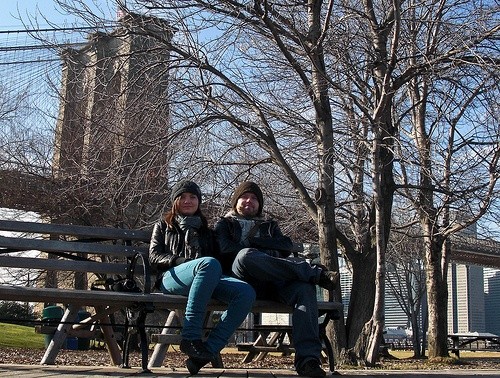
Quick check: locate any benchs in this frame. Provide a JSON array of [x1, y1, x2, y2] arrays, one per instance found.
[[0, 217, 342, 378], [445, 329, 497, 355], [237, 316, 309, 365]]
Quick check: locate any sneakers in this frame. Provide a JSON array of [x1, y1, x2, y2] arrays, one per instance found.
[[320, 268, 340, 290], [298, 360, 326, 378], [180, 339, 215, 360], [185, 357, 210, 376]]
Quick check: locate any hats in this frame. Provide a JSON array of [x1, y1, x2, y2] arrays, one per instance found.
[[232, 181, 263, 212], [172, 181, 201, 202]]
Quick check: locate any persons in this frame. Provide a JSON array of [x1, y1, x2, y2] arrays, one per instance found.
[[214, 181, 341, 378], [148, 179, 257, 377]]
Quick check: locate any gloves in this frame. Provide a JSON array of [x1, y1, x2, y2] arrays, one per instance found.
[[174, 257, 190, 264]]
